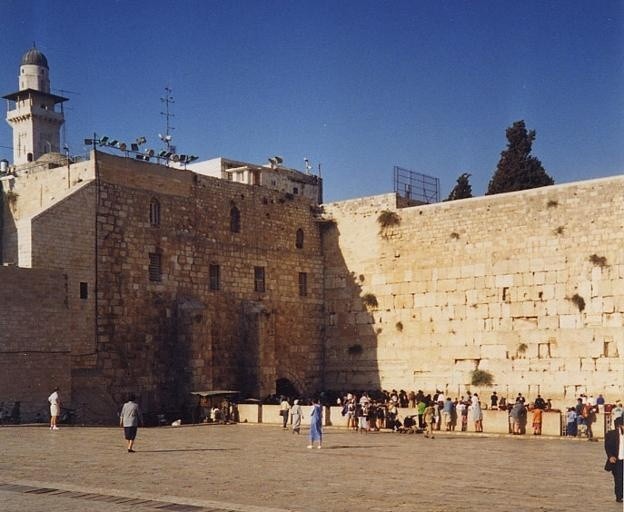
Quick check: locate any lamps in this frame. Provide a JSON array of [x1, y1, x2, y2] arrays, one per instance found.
[[84, 136, 190, 164]]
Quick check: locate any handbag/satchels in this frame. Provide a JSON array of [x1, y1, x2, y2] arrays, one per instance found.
[[280, 410, 283, 416]]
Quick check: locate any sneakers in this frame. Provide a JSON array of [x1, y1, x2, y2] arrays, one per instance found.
[[50, 427, 60, 430], [306, 446, 322, 449], [128, 449, 135, 452]]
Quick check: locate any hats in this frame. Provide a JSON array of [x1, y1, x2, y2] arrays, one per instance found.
[[294, 400, 299, 405]]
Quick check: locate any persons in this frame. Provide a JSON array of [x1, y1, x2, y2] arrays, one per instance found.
[[264, 393, 304, 434], [490, 391, 546, 435], [200, 395, 240, 425], [603, 416, 623, 503], [47, 386, 61, 430], [567, 397, 596, 441], [580, 393, 604, 409], [606, 402, 623, 429], [0, 401, 72, 424], [306, 398, 322, 449], [118, 394, 143, 453], [313, 390, 483, 439]]
